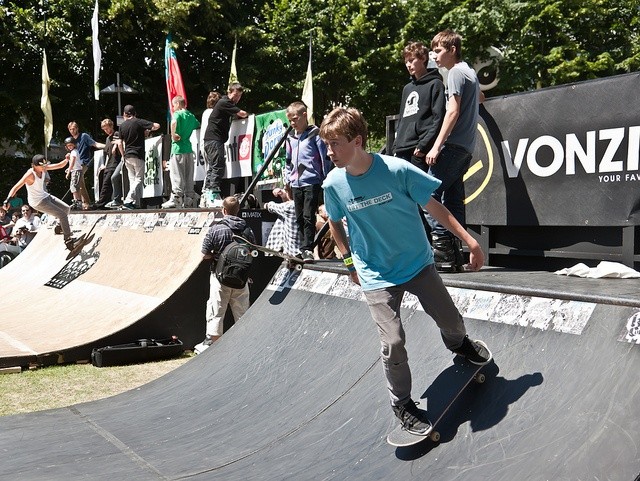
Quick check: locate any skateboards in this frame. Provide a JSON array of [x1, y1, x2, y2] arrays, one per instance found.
[[386, 340, 489, 447], [231, 235, 305, 271], [65, 239, 86, 260], [99, 168, 105, 198], [119, 162, 130, 203], [434, 260, 465, 273]]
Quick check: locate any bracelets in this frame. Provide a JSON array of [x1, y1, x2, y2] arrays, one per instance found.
[[346, 267, 357, 274], [339, 251, 353, 265]]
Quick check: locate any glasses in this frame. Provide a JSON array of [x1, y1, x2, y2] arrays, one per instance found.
[[22, 210, 29, 212]]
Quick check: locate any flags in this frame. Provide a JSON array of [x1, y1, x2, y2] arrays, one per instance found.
[[89, 0, 102, 101], [225, 40, 240, 95], [163, 31, 189, 122], [297, 42, 315, 126], [38, 47, 55, 147]]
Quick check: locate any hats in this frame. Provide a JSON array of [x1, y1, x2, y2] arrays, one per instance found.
[[32, 154, 51, 166], [124, 105, 136, 116]]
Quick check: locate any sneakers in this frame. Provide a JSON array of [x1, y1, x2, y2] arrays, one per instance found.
[[209, 193, 223, 208], [194, 340, 209, 353], [198, 193, 205, 208], [301, 249, 314, 262], [160, 199, 182, 209], [54, 225, 62, 234], [390, 400, 432, 435], [184, 195, 198, 208], [65, 233, 86, 252], [454, 337, 493, 366], [122, 203, 135, 211], [104, 200, 120, 208]]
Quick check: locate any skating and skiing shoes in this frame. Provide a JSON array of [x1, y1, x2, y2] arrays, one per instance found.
[[430, 228, 456, 272], [452, 236, 465, 272], [69, 199, 81, 211]]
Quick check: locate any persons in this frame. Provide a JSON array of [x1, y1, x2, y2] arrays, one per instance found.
[[282, 100, 332, 263], [201, 195, 258, 346], [0, 225, 9, 242], [117, 105, 160, 211], [40, 212, 48, 225], [319, 104, 485, 435], [160, 94, 201, 209], [89, 118, 119, 209], [419, 28, 485, 264], [9, 204, 40, 252], [11, 211, 21, 223], [0, 206, 15, 236], [104, 136, 130, 210], [65, 121, 106, 211], [198, 91, 222, 208], [6, 192, 23, 217], [342, 216, 350, 246], [203, 81, 249, 207], [0, 238, 22, 268], [312, 204, 328, 260], [390, 38, 446, 245], [261, 187, 302, 260], [2, 152, 86, 252], [66, 136, 84, 211]]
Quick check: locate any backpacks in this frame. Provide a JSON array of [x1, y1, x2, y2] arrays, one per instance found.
[[216, 241, 253, 289]]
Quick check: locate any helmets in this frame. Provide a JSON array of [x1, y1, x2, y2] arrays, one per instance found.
[[64, 137, 77, 146]]
